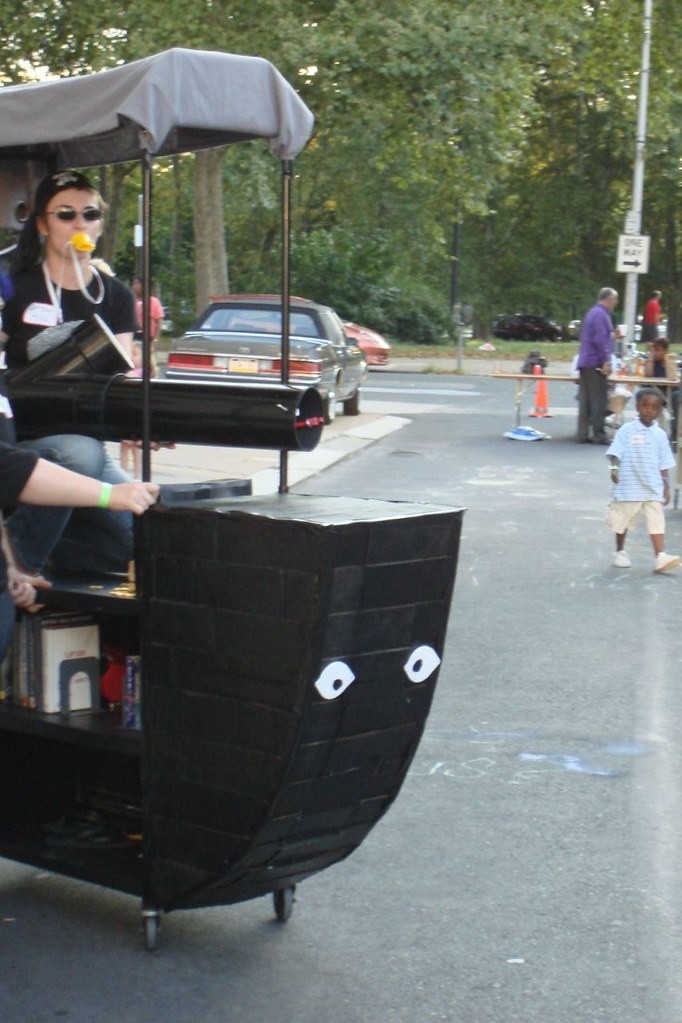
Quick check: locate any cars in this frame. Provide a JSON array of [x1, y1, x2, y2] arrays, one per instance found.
[[212, 295, 389, 368], [493, 313, 559, 342], [165, 299, 369, 427]]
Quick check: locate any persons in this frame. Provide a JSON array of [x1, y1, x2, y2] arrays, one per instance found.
[[605, 387, 681, 573], [641, 338, 679, 407], [640, 290, 668, 342], [571, 287, 619, 446], [0, 171, 136, 662], [0, 440, 160, 613], [129, 276, 164, 379]]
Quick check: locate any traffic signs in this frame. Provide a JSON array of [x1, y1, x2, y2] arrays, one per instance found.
[[614, 234, 650, 274]]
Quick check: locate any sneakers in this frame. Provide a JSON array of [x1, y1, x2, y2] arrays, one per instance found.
[[653, 552, 681, 573], [613, 550, 631, 568]]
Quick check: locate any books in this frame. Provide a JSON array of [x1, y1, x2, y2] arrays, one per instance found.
[[123, 656, 141, 731], [10, 614, 99, 713]]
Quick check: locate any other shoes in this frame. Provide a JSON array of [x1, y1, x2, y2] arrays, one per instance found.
[[593, 436, 612, 445], [578, 437, 592, 443]]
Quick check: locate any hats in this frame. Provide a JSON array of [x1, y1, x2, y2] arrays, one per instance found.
[[33, 170, 93, 214]]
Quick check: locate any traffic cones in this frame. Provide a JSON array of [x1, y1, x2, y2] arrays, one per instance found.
[[528, 378, 554, 418]]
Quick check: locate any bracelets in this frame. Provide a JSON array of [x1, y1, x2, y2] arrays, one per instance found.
[[605, 363, 612, 366], [608, 464, 619, 470], [97, 483, 111, 507]]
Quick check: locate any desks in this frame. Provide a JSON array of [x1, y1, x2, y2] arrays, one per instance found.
[[492, 374, 680, 453]]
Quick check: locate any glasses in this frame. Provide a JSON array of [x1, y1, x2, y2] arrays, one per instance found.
[[42, 208, 102, 222]]
